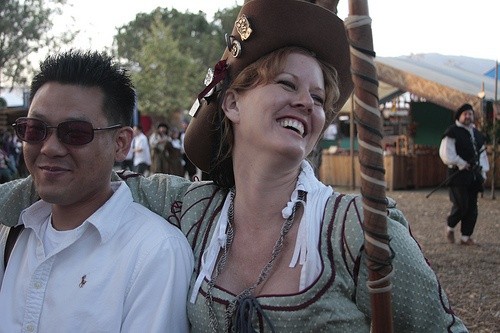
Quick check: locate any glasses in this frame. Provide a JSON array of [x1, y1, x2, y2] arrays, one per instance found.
[[12, 117, 122, 145]]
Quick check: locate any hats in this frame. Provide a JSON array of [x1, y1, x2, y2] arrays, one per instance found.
[[183, 0, 353, 176], [456, 104, 472, 120]]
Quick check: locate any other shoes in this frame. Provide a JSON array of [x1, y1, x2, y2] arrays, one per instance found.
[[446, 231, 454, 244], [461, 239, 480, 247]]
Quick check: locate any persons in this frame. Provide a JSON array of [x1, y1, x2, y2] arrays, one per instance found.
[[439, 104, 489, 246], [149, 123, 191, 178], [0, 0, 469, 333], [0, 51, 195, 333], [121, 125, 151, 170]]
